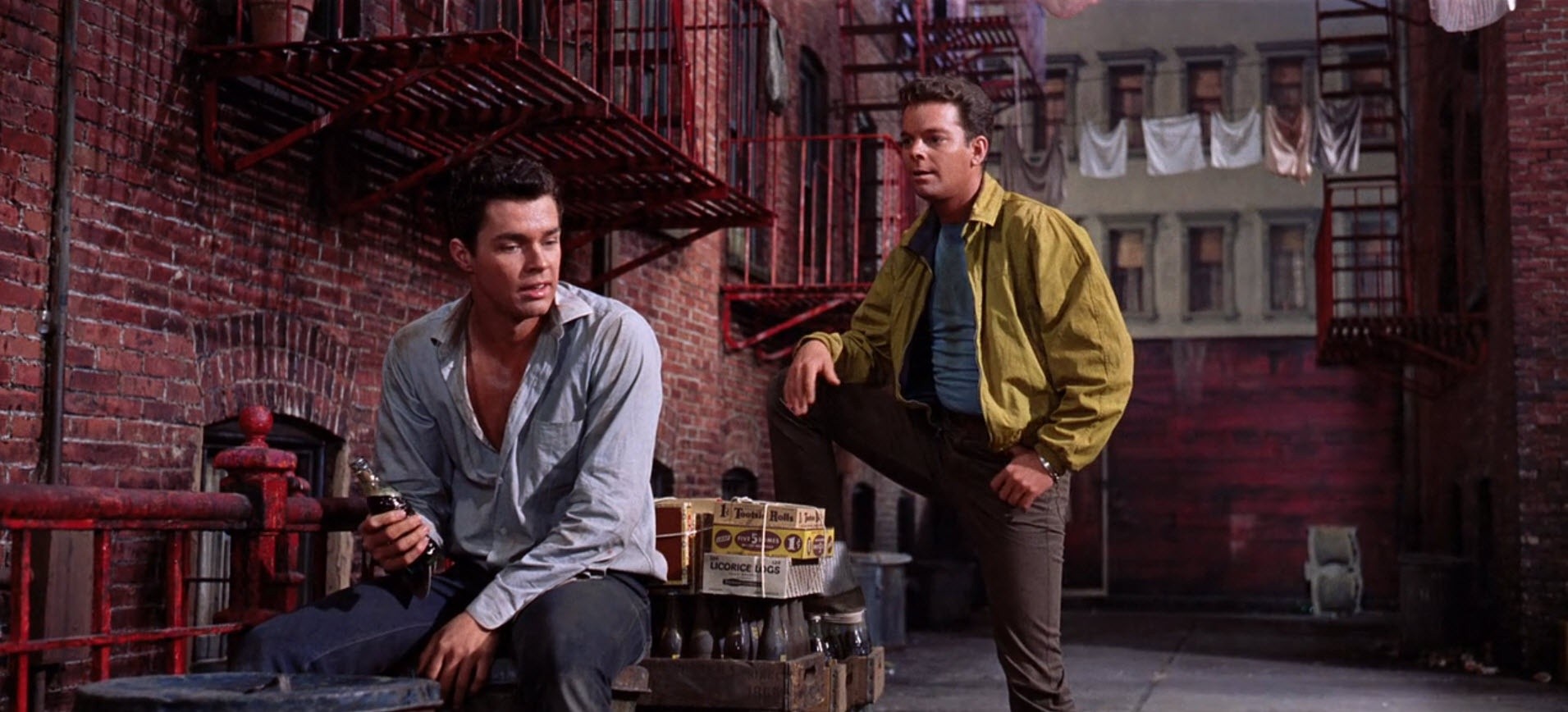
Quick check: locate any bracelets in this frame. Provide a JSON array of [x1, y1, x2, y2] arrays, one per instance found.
[[1037, 454, 1058, 481]]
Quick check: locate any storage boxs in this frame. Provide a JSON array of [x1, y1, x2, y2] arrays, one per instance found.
[[636, 646, 885, 712], [655, 497, 836, 595]]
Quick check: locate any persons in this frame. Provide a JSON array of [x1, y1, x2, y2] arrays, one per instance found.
[[222, 155, 667, 712], [650, 458, 675, 498], [766, 78, 1134, 712], [721, 466, 757, 502]]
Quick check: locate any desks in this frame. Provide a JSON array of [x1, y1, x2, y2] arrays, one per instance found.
[[660, 539, 857, 643]]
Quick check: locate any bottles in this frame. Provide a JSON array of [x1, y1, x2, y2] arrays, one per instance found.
[[650, 597, 872, 663], [349, 456, 449, 575]]
